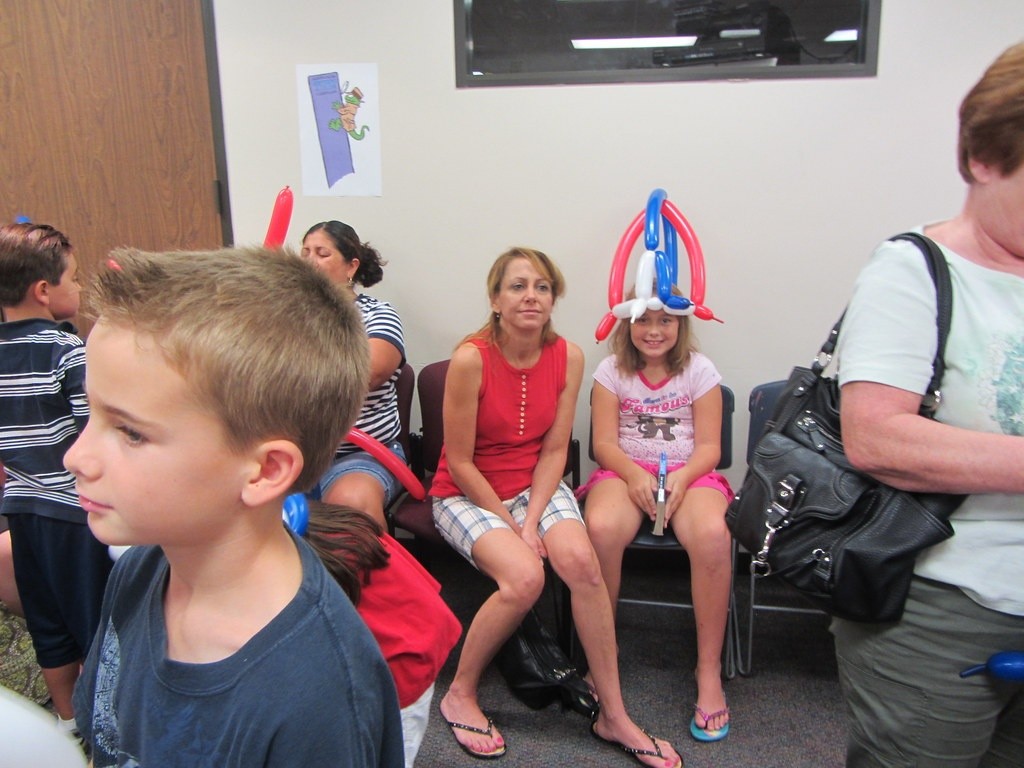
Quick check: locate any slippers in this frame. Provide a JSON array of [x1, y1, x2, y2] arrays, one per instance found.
[[438, 699, 506, 759], [590, 721, 683, 768], [690, 688, 728, 742]]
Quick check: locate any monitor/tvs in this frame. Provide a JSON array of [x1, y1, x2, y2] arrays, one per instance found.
[[454, 0, 884, 88]]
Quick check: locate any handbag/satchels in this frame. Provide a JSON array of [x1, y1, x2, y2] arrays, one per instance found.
[[723, 233, 969, 626]]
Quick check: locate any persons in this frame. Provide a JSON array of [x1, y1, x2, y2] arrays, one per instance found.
[[564, 278, 732, 743], [280, 497, 464, 768], [300, 221, 405, 535], [63, 247, 405, 767], [428, 245, 688, 768], [832, 42, 1022, 768], [0, 223, 122, 749], [0, 515, 23, 616]]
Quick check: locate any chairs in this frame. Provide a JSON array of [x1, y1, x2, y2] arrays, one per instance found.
[[732, 382, 831, 674], [568, 374, 736, 678], [387, 361, 582, 661], [385, 365, 415, 541]]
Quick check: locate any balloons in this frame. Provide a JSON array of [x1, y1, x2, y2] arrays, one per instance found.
[[959, 651, 1023, 686], [342, 427, 428, 505], [262, 185, 293, 253], [595, 188, 725, 343], [106, 258, 122, 271]]
